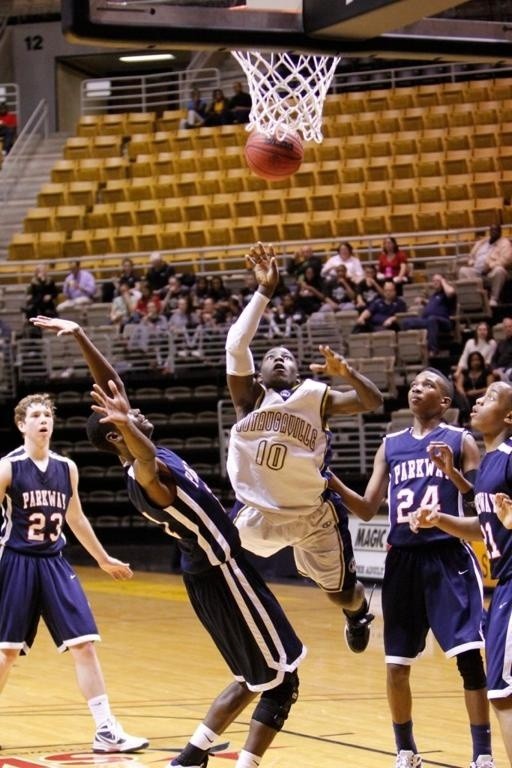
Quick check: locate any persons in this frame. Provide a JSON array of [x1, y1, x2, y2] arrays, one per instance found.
[[326, 365, 495, 768], [1, 101, 18, 155], [407, 380, 512, 766], [28, 312, 311, 768], [17, 219, 511, 429], [220, 240, 384, 655], [0, 389, 152, 759], [179, 82, 254, 130]]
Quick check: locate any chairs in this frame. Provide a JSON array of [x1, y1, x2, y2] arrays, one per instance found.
[[0, 77, 511, 543]]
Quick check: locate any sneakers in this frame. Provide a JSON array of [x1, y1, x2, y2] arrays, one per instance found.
[[395, 751, 421, 767], [91, 717, 148, 754], [342, 601, 375, 652]]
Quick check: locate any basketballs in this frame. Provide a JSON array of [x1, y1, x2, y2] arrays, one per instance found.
[[244, 122, 303, 181]]
